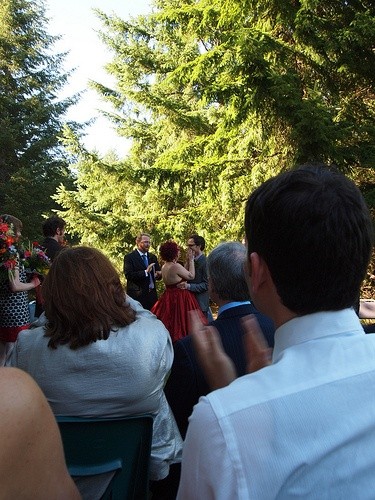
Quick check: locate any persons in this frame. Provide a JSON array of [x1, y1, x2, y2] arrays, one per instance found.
[[174, 164, 375, 500], [0, 211, 279, 500]]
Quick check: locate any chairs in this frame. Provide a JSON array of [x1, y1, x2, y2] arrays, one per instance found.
[[55, 414, 154, 500]]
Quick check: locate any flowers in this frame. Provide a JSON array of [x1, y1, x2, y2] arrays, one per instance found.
[[20, 240, 52, 305], [0, 215, 24, 289]]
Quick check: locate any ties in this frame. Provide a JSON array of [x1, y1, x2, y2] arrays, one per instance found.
[[142, 255, 154, 289]]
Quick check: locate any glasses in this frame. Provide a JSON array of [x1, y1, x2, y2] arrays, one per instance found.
[[187, 244, 196, 247]]
